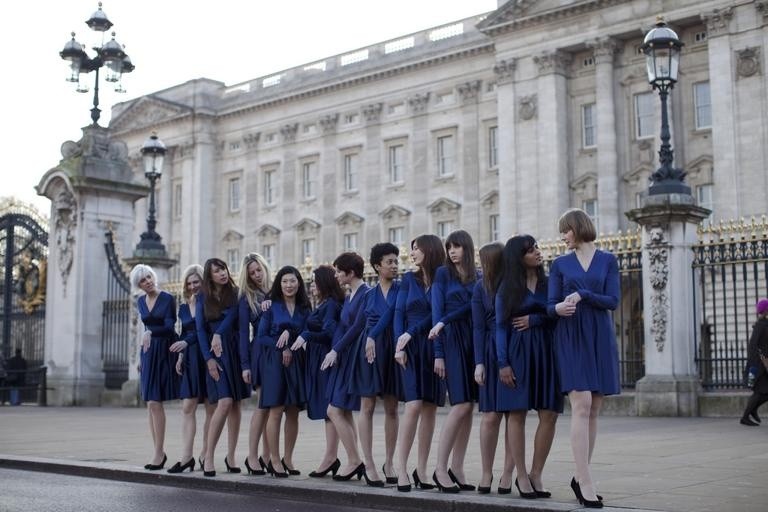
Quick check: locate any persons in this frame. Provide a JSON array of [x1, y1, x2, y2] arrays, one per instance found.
[[342, 241, 400, 487], [495, 235, 565, 499], [0, 346, 27, 406], [471, 241, 516, 495], [128, 263, 180, 472], [428, 231, 482, 494], [166, 264, 217, 474], [741, 299, 768, 427], [258, 265, 312, 479], [291, 266, 346, 479], [547, 208, 622, 508], [237, 253, 273, 476], [195, 257, 244, 477], [319, 250, 373, 481], [392, 234, 444, 491]]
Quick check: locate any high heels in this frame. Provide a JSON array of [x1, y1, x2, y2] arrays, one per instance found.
[[309, 458, 411, 492], [571, 477, 603, 508], [413, 469, 551, 499], [167, 457, 216, 476], [225, 456, 241, 473], [245, 456, 300, 477], [144, 454, 166, 470]]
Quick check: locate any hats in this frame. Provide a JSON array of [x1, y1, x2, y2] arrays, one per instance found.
[[756, 298, 768, 313]]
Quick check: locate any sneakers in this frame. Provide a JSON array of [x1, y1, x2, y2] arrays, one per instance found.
[[741, 413, 761, 426]]
